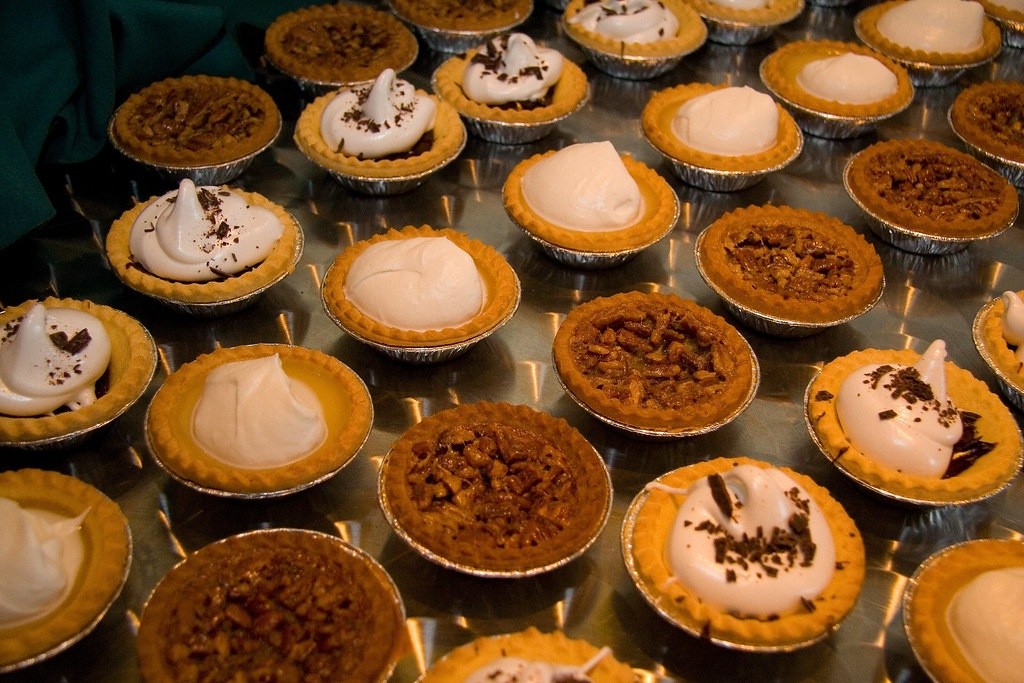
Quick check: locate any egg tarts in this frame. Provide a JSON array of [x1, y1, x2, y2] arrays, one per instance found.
[[0, 0, 1024, 683]]
